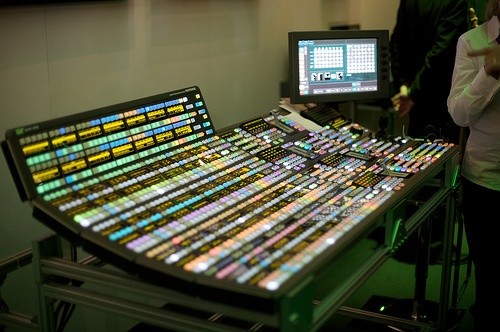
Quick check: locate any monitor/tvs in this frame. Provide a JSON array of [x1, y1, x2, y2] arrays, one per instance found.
[[287, 30, 390, 103]]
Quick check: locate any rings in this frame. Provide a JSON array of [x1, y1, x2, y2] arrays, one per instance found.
[[492, 58, 497, 63]]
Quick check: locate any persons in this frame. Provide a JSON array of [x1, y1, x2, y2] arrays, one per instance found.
[[391, 0, 468, 265], [447, 0, 500, 332]]
